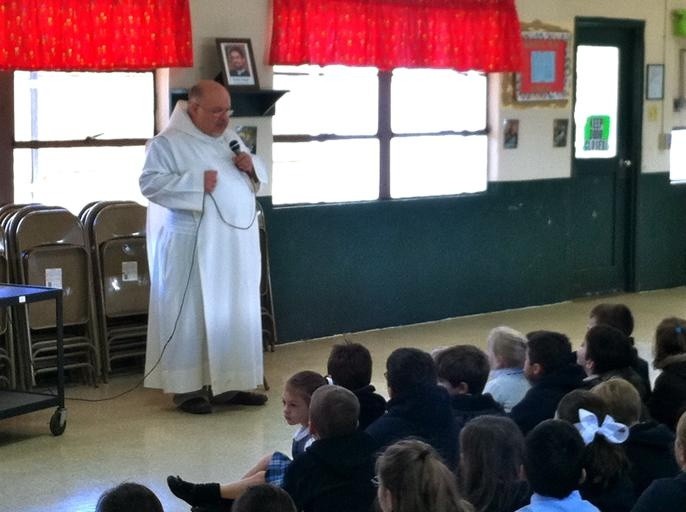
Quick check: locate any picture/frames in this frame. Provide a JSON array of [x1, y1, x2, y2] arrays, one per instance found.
[[515, 31, 573, 102], [645, 63, 665, 101], [553, 118, 569, 147], [502, 119, 519, 149], [214, 38, 260, 90]]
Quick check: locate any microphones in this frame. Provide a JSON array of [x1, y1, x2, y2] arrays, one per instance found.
[[229, 140, 260, 183]]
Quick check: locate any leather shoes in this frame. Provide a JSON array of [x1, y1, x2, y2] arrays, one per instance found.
[[179, 398, 212, 413], [227, 392, 268, 405]]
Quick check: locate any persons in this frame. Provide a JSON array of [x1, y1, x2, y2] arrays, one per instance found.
[[327, 338, 387, 431], [233, 481, 296, 511], [632, 410, 686, 512], [435, 341, 504, 413], [482, 326, 533, 413], [515, 419, 601, 512], [283, 385, 377, 512], [139, 78, 269, 414], [460, 417, 529, 512], [555, 389, 634, 511], [589, 377, 677, 506], [646, 317, 686, 434], [372, 437, 475, 512], [228, 47, 250, 76], [167, 370, 335, 511], [510, 330, 588, 436], [364, 349, 458, 476], [96, 480, 163, 512], [587, 303, 652, 404], [577, 324, 646, 406]]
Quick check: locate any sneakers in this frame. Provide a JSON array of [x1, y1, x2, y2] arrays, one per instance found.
[[167, 475, 234, 512]]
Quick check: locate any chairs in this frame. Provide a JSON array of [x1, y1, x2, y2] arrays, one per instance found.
[[0, 200, 277, 392]]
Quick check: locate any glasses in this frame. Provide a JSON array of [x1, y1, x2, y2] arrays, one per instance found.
[[371, 476, 382, 487], [231, 56, 242, 60], [196, 104, 234, 118]]
